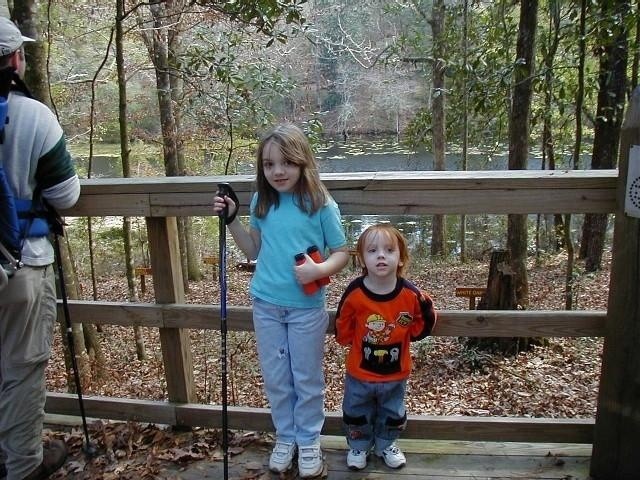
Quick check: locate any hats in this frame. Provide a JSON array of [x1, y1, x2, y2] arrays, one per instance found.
[[0, 17, 36, 56]]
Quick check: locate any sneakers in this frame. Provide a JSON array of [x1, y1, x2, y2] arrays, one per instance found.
[[347, 444, 406, 469], [269, 441, 324, 478], [0, 439, 67, 479]]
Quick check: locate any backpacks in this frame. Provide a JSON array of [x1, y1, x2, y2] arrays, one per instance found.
[[1, 66, 47, 289]]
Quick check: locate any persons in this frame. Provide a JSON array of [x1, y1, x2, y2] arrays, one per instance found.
[[213, 124, 350, 480], [335, 224, 436, 470], [0, 17, 81, 480]]
